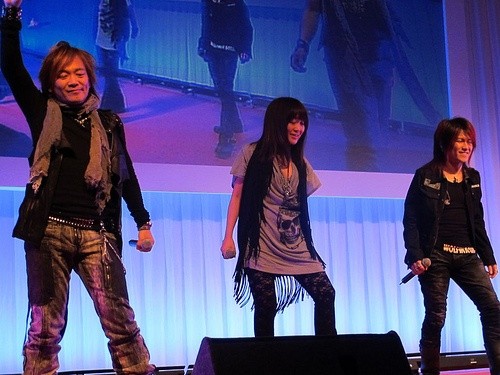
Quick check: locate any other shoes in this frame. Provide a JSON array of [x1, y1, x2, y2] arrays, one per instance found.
[[214, 117, 243, 133], [215, 132, 234, 160]]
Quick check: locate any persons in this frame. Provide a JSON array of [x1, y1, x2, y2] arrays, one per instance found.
[[402, 116, 500, 375], [0, 0, 163, 374], [88, 0, 140, 115], [287, 0, 392, 172], [191, 0, 254, 159], [221, 96, 336, 338]]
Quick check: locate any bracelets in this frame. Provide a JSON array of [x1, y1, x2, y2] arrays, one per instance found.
[[138, 220, 152, 232], [296, 39, 310, 52], [0, 6, 23, 22]]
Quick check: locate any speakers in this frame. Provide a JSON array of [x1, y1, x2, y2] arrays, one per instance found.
[[192, 330, 412, 375]]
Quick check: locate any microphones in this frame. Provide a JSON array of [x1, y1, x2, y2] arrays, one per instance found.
[[128, 240, 152, 249], [226, 249, 235, 258], [399, 258, 431, 285]]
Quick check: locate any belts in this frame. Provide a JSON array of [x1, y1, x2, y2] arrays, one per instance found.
[[443, 243, 477, 255], [48, 211, 111, 232]]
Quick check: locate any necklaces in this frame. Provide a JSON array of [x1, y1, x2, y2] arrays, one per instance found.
[[444, 167, 460, 184], [275, 151, 292, 199]]
